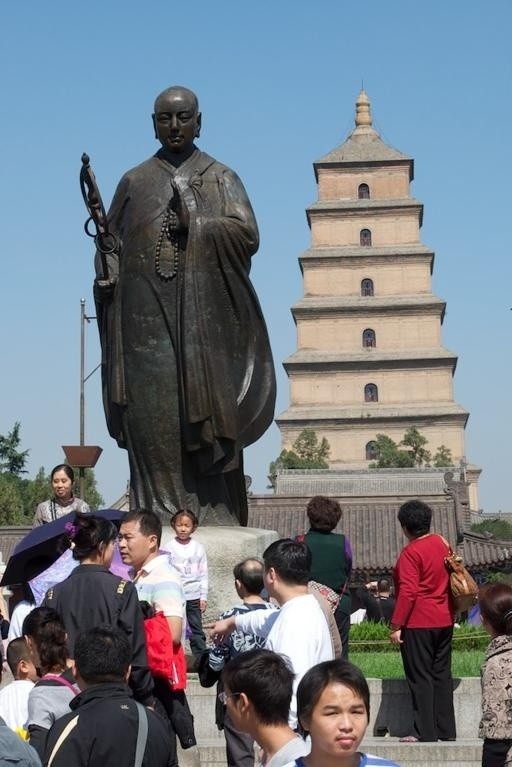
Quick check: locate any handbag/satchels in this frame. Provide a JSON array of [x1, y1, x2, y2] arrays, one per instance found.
[[444, 551, 480, 612], [307, 580, 341, 613]]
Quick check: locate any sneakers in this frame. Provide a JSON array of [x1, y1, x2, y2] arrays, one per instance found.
[[399, 736, 419, 742]]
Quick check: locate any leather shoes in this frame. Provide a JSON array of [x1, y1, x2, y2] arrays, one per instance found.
[[444, 737, 456, 741]]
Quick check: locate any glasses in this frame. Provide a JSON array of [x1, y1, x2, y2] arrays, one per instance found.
[[218, 691, 240, 703]]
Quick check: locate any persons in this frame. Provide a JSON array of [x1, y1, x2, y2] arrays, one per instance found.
[[159, 509, 208, 669], [279, 660, 398, 767], [475, 579, 512, 765], [2, 506, 186, 767], [30, 464, 91, 529], [387, 499, 457, 741], [201, 538, 347, 767], [91, 82, 274, 529], [347, 572, 490, 629], [291, 491, 358, 659]]
